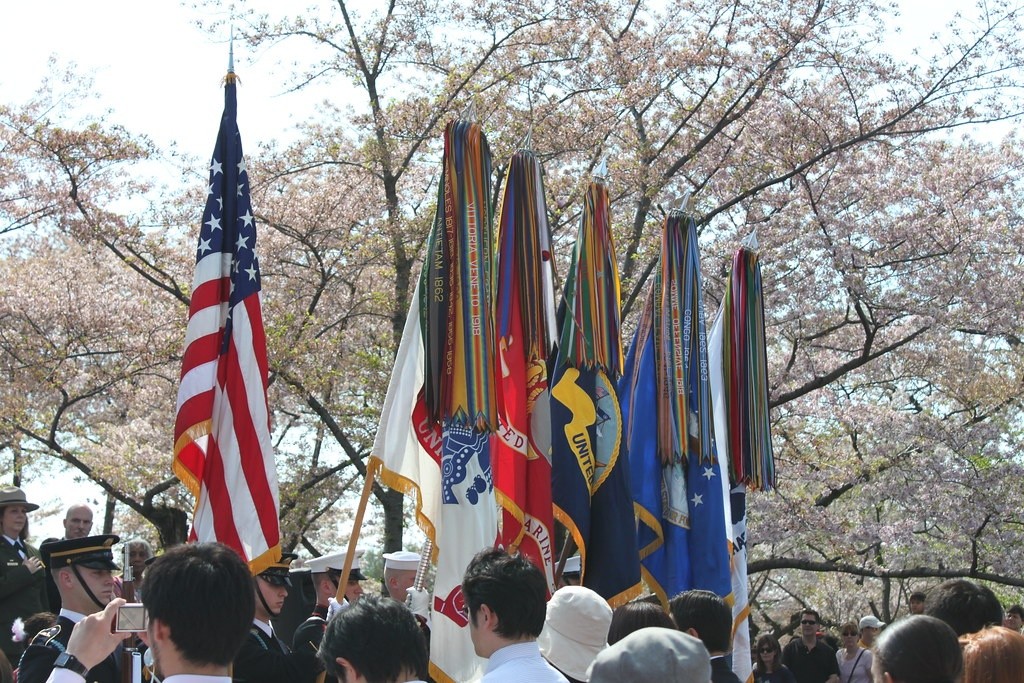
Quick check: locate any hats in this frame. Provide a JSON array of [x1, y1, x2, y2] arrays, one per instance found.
[[382, 551, 421, 570], [304, 549, 367, 580], [555, 556, 581, 575], [0, 486, 39, 512], [860, 616, 887, 630], [587, 628, 711, 683], [41, 535, 121, 571], [537, 585, 613, 682], [258, 553, 298, 587]]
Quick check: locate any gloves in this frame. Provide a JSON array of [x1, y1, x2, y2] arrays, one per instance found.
[[325, 596, 350, 622], [404, 586, 428, 622]]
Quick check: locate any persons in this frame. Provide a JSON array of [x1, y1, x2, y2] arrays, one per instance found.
[[0, 479, 1024, 683]]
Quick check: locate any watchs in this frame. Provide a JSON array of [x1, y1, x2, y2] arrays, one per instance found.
[[52, 652, 89, 679]]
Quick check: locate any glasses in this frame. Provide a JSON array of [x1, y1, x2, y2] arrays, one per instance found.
[[461, 604, 495, 614], [759, 648, 776, 653], [843, 632, 858, 636], [800, 620, 816, 625]]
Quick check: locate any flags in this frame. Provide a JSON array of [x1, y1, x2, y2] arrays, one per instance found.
[[362, 118, 775, 683], [172, 69, 280, 576]]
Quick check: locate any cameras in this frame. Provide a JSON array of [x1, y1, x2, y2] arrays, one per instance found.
[[111, 602, 150, 632]]
